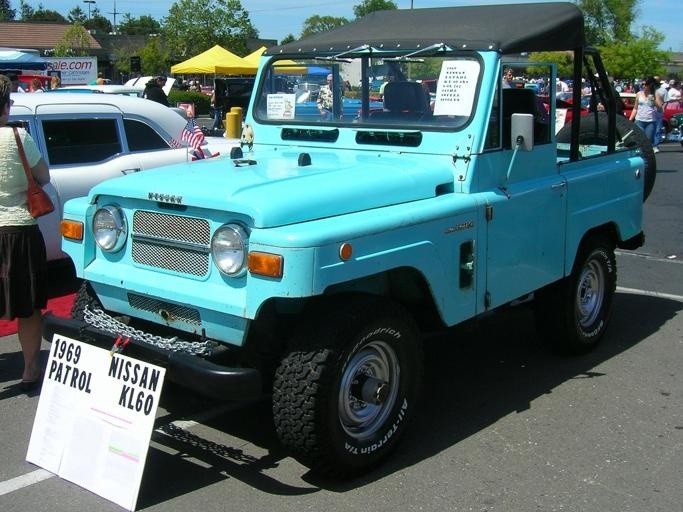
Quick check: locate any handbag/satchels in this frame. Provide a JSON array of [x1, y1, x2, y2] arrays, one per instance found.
[[12, 127, 55, 218]]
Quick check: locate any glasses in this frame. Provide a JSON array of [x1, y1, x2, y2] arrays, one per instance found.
[[5, 97, 14, 106]]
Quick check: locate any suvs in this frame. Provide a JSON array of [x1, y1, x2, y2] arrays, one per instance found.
[[42, 2, 656, 481]]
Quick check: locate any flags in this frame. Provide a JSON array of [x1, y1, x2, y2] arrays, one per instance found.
[[179, 116, 204, 150]]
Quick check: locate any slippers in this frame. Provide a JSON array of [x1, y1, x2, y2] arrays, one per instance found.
[[21, 369, 43, 390]]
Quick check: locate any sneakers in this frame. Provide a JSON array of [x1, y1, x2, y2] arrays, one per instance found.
[[654, 146, 660, 154]]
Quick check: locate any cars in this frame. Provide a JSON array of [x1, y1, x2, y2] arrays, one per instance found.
[[543, 91, 683, 144], [5, 75, 241, 266]]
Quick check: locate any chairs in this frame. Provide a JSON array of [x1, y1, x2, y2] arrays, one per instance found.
[[498, 87, 538, 137], [379, 80, 427, 123]]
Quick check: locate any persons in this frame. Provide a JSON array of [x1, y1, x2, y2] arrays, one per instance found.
[[143, 74, 171, 107], [378, 78, 390, 104], [501, 67, 683, 155], [49, 75, 60, 90], [96, 71, 108, 85], [6, 73, 25, 94], [0, 74, 51, 392], [175, 77, 201, 93], [316, 72, 345, 121], [32, 78, 47, 93], [209, 90, 224, 130]]
